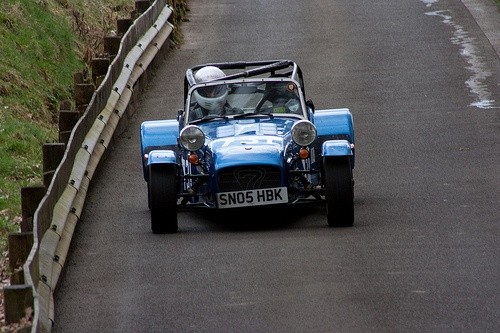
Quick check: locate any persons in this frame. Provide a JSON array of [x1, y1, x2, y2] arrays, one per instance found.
[[260, 74, 303, 115], [189, 65, 244, 122]]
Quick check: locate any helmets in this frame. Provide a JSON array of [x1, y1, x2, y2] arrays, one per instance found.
[[194, 66, 228, 110]]
